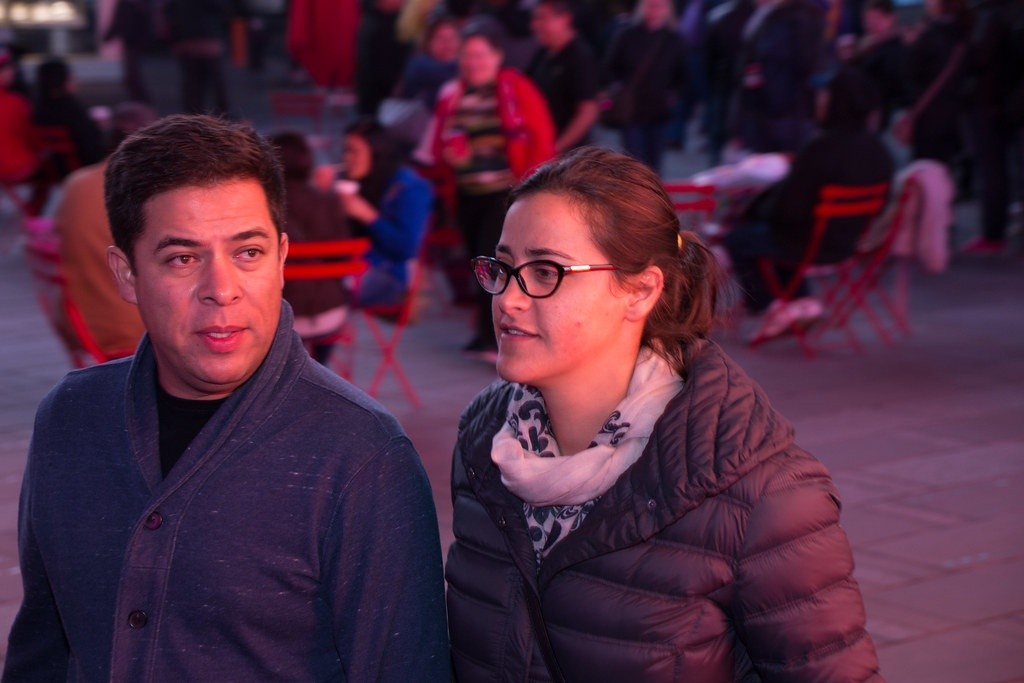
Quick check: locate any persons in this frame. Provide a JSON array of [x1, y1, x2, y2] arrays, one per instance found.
[[446, 150, 886, 683], [0, 0, 1024, 370], [0, 114, 449, 683]]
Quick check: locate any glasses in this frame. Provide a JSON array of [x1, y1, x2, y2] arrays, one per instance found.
[[470, 249, 615, 298]]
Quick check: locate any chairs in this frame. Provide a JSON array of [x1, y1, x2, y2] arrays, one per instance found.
[[0, 93, 916, 406]]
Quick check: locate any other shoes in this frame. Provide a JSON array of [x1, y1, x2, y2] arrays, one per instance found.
[[462, 335, 498, 363], [737, 294, 828, 346]]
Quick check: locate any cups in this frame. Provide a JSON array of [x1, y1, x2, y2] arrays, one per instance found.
[[333, 179, 360, 195]]
